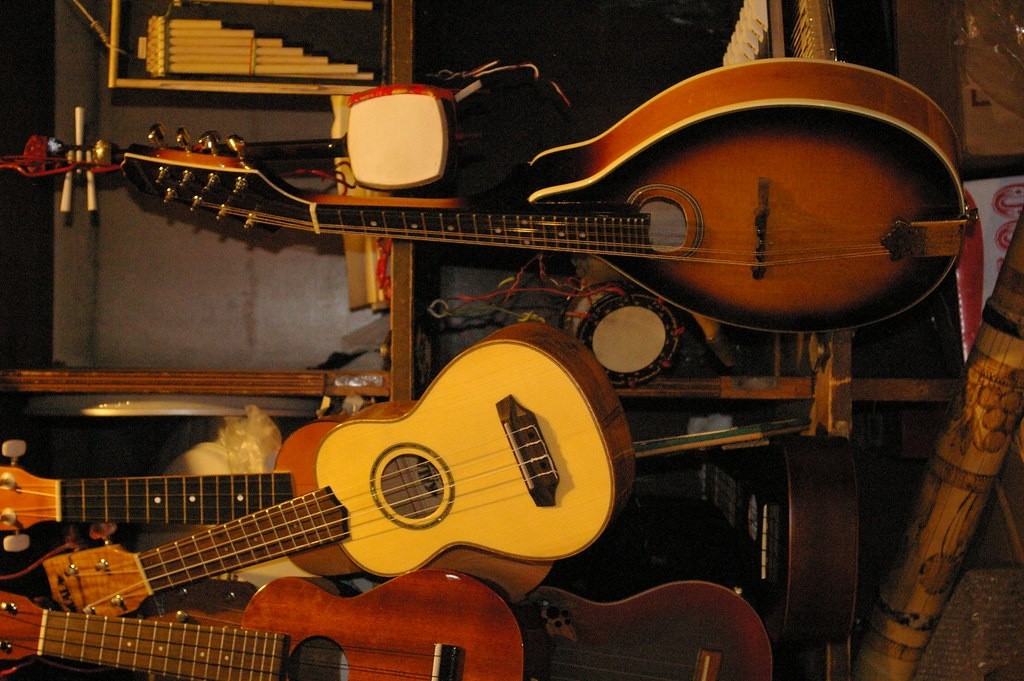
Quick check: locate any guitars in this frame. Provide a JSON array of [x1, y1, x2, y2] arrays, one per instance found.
[[40, 317, 639, 620], [0, 396, 557, 603], [1, 566, 556, 680], [140, 576, 778, 681], [117, 50, 968, 336], [1, 99, 349, 233]]
